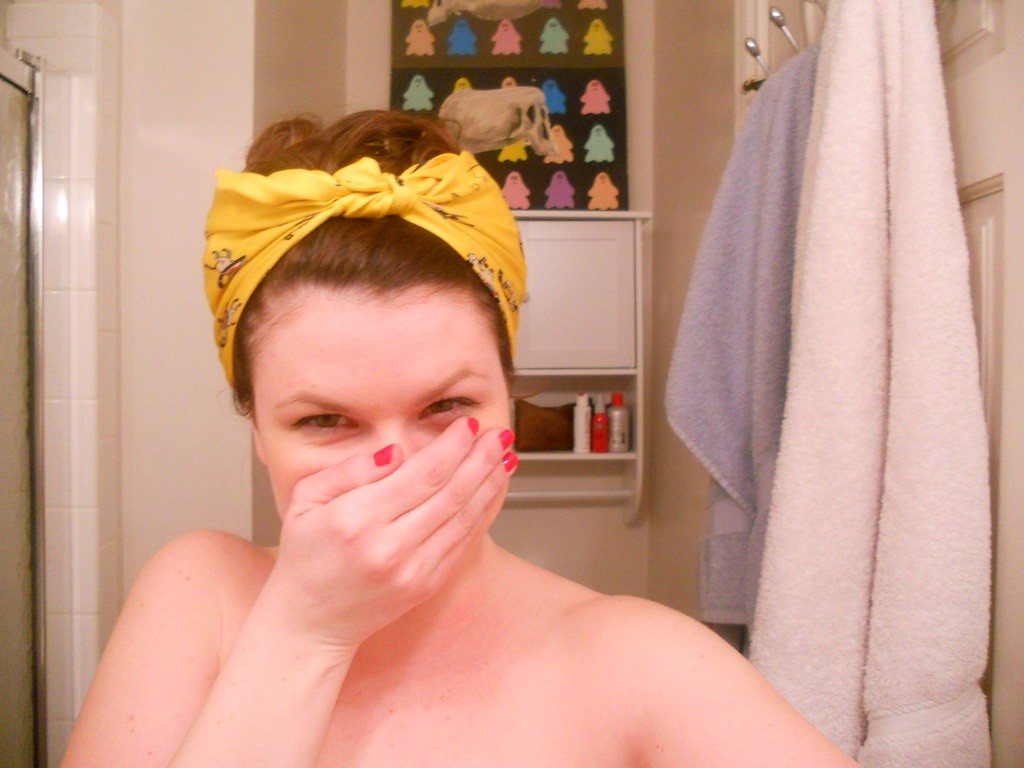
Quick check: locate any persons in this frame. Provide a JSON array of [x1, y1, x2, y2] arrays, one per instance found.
[[56, 109, 860, 768]]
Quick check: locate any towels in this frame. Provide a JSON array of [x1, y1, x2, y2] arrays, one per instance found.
[[666, 45, 819, 623], [749, 2, 991, 768]]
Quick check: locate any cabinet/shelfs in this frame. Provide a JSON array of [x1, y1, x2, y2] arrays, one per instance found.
[[508, 211, 655, 527]]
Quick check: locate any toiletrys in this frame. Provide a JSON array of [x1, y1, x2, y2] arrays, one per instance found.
[[591, 393, 608, 453], [607, 392, 629, 453], [573, 390, 591, 454]]
